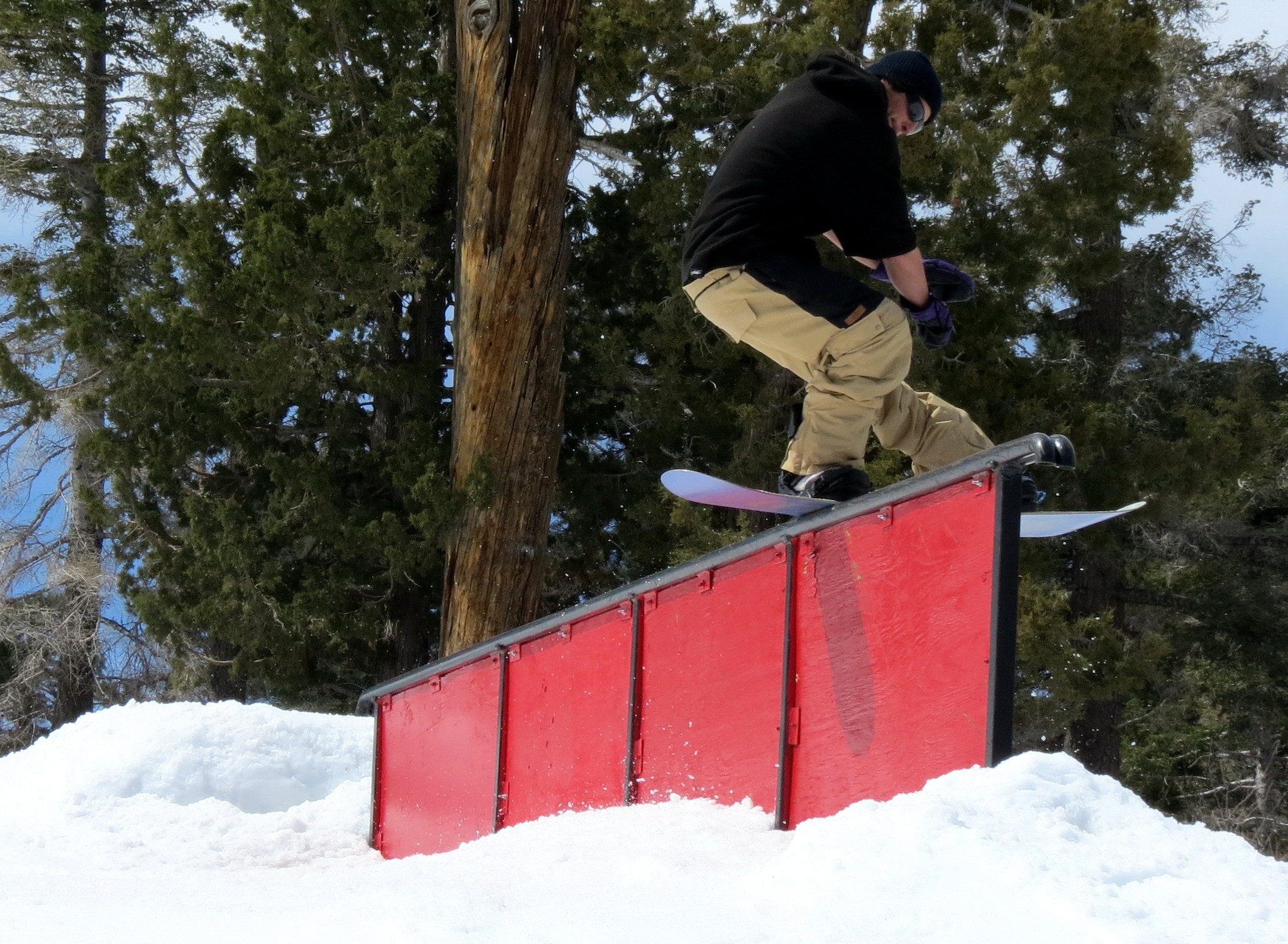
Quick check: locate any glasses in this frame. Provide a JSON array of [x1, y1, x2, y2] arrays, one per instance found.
[[903, 91, 925, 136]]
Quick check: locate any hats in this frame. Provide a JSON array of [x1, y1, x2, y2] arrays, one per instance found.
[[865, 51, 943, 126]]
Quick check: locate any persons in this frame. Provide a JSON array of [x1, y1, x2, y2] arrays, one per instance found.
[[682, 49, 1040, 514]]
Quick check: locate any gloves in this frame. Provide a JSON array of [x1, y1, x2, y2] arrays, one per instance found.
[[869, 259, 974, 302], [899, 286, 952, 349]]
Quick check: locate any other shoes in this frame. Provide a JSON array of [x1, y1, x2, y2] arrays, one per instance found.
[[785, 464, 870, 502], [1004, 476, 1037, 512]]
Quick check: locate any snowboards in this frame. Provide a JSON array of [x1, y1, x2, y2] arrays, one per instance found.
[[658, 469, 1147, 539]]
